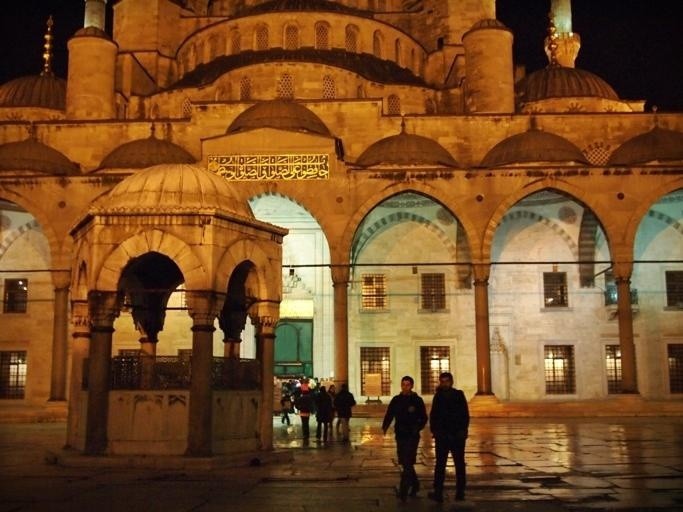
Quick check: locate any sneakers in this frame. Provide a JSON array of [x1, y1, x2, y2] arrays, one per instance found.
[[401, 484, 465, 503]]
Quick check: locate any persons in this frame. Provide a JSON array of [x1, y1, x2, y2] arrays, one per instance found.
[[427, 372, 469, 503], [381, 375, 428, 507], [279, 377, 356, 443]]
